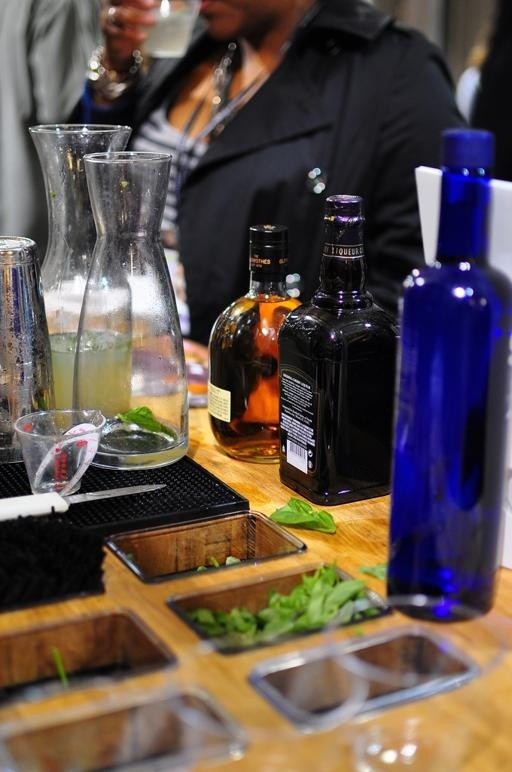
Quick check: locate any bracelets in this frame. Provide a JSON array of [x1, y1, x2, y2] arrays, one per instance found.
[[82, 43, 143, 100]]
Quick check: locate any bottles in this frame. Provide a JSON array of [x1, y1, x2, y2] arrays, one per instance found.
[[277, 194, 397, 507], [386, 127, 512, 624], [207, 223, 305, 465], [72, 150, 190, 471], [26, 124, 133, 435]]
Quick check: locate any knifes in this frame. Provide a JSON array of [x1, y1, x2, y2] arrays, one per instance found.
[[0, 481, 167, 521]]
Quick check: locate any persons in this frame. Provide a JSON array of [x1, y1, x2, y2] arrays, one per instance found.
[[0, 1, 86, 264], [68, 2, 472, 380]]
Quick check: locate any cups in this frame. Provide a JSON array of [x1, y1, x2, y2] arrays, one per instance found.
[[15, 409, 105, 495], [0, 232, 55, 464], [136, 0, 203, 59], [160, 634, 371, 772], [330, 595, 503, 771]]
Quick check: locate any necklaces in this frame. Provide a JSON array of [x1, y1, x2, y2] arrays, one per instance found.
[[174, 23, 298, 226]]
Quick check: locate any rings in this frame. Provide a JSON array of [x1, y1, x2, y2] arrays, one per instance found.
[[105, 7, 123, 21]]
